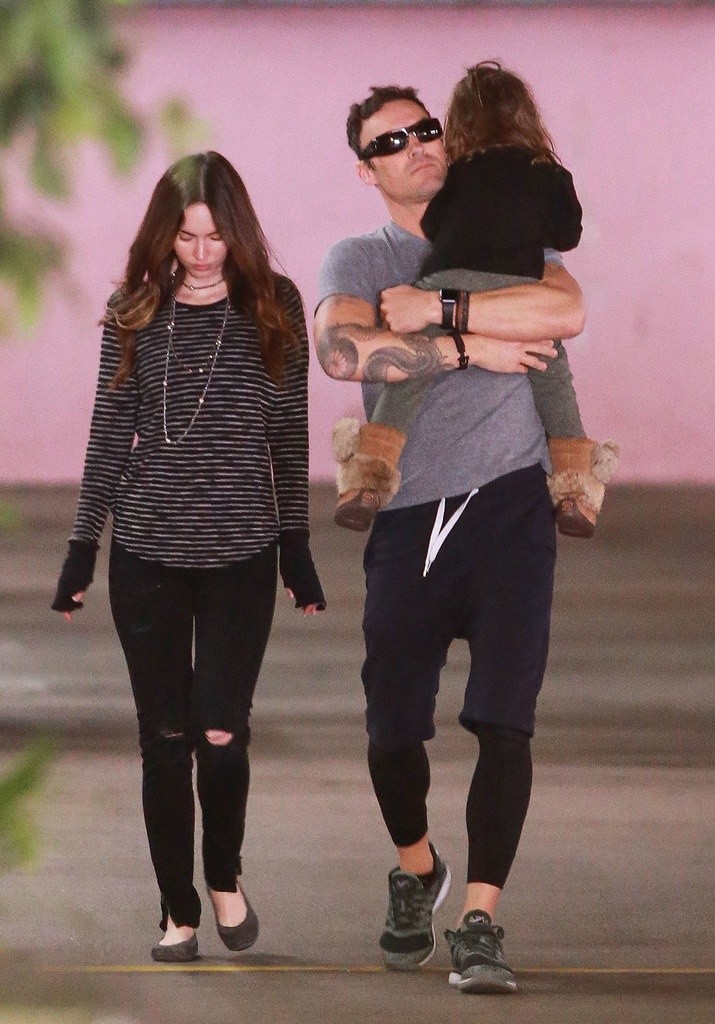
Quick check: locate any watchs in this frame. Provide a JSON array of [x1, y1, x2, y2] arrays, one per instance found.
[[439, 288, 456, 330]]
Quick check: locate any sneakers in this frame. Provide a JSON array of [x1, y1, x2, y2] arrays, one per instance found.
[[379, 841, 451, 973], [443, 909, 517, 993]]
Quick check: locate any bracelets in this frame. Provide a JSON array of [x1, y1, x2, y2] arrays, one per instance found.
[[446, 331, 470, 371], [455, 289, 470, 333]]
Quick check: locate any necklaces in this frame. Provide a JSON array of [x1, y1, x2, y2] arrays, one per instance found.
[[162, 279, 230, 441]]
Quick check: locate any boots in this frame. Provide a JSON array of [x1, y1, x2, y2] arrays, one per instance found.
[[546, 438, 620, 539], [332, 417, 406, 531]]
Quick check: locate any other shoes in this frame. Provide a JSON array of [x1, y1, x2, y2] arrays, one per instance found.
[[151, 933, 199, 962], [207, 882, 259, 951]]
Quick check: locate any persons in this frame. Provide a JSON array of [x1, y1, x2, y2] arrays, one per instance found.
[[46, 145, 330, 960], [334, 60, 619, 538], [313, 85, 587, 995]]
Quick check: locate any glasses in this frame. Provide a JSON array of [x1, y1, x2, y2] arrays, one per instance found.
[[361, 118, 444, 162]]
